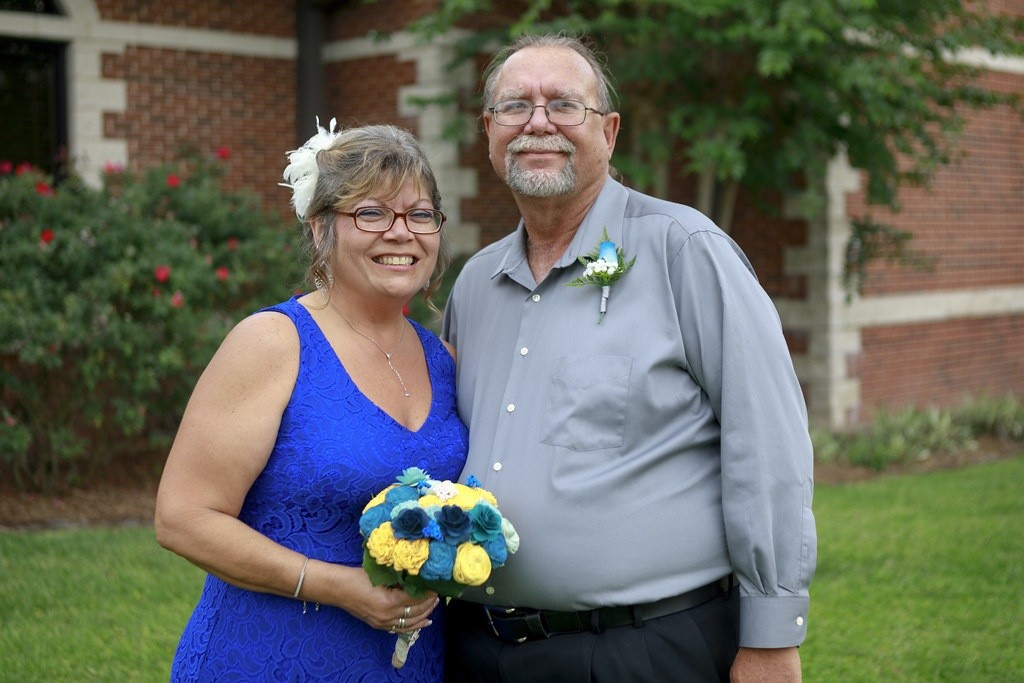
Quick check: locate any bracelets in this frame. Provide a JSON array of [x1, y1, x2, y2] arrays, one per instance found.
[[294, 557, 309, 597]]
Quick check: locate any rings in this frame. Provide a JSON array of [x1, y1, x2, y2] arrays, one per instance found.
[[398, 618, 405, 629], [404, 606, 411, 619], [392, 626, 395, 631], [389, 630, 395, 634]]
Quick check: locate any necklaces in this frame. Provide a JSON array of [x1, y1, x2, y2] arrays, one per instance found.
[[321, 286, 410, 398]]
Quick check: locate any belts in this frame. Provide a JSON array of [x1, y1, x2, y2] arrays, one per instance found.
[[479, 571, 740, 644]]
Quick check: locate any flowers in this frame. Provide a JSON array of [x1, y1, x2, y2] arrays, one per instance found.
[[560, 226, 637, 324], [275, 115, 336, 223], [358, 467, 520, 669]]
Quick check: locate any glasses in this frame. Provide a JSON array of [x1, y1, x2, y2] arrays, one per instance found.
[[489, 97, 604, 128], [316, 204, 449, 234]]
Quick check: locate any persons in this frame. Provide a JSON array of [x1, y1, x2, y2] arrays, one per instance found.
[[153, 116, 471, 683], [437, 29, 818, 683]]
